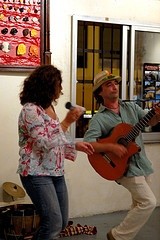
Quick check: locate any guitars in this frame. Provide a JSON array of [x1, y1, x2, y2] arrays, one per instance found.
[[87, 101, 160, 181]]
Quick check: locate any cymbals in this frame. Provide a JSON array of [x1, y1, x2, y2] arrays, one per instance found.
[[2, 182, 26, 198]]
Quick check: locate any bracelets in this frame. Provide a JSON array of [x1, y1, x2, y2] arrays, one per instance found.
[[61, 122, 69, 128]]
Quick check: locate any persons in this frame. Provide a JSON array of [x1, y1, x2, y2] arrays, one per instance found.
[[16, 64, 94, 240], [83, 70, 160, 240]]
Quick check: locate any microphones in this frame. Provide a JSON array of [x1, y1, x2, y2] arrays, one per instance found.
[[65, 102, 76, 110]]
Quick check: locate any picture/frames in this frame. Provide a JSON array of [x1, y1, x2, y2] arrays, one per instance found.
[[0, 0, 51, 72]]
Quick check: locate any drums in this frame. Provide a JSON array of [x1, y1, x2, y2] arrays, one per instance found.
[[0, 204, 42, 236]]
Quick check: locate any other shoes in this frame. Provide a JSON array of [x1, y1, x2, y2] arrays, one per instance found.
[[106, 230, 116, 240]]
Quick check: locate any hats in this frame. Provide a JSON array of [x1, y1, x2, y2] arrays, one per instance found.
[[92, 70, 121, 94]]
